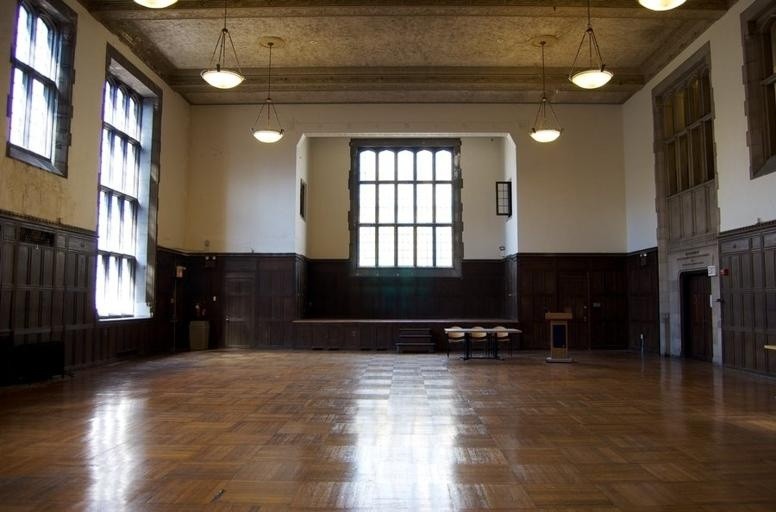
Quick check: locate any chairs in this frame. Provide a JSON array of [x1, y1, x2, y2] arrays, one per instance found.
[[447, 325, 512, 359]]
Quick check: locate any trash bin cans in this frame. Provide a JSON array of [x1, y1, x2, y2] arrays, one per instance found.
[[188, 320, 210, 350]]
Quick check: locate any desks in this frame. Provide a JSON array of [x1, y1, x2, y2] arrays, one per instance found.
[[443, 328, 522, 359]]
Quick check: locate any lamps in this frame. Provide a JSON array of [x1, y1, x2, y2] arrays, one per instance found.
[[135, 0, 285, 144], [528, 0, 685, 144]]
